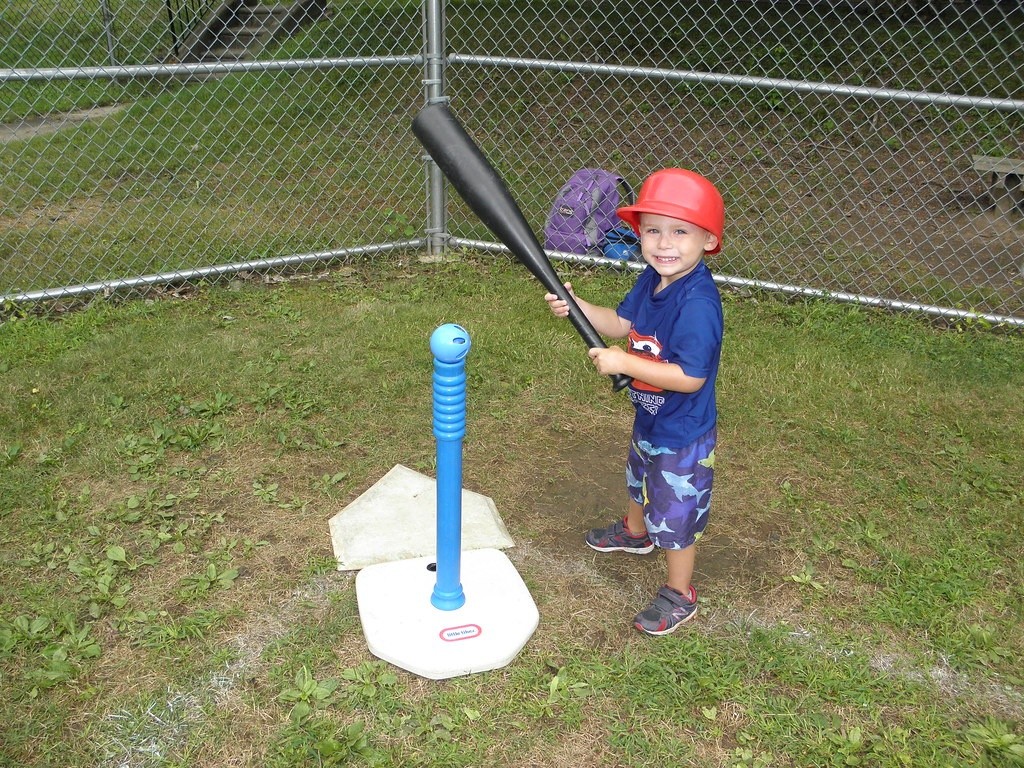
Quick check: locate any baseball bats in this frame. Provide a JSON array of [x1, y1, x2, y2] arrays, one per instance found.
[[411, 102, 635, 393]]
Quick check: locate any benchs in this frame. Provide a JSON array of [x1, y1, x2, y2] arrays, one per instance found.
[[971, 154, 1024, 219]]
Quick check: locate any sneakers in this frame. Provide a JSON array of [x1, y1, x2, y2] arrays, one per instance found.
[[633, 585, 698, 636], [585, 515, 654, 554]]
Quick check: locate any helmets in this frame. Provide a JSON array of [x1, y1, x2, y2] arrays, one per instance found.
[[616, 168, 724, 255]]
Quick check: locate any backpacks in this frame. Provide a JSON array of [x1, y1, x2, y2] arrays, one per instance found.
[[544, 168, 635, 256]]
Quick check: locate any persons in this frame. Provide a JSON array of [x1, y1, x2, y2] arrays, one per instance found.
[[545, 168, 724, 637]]
